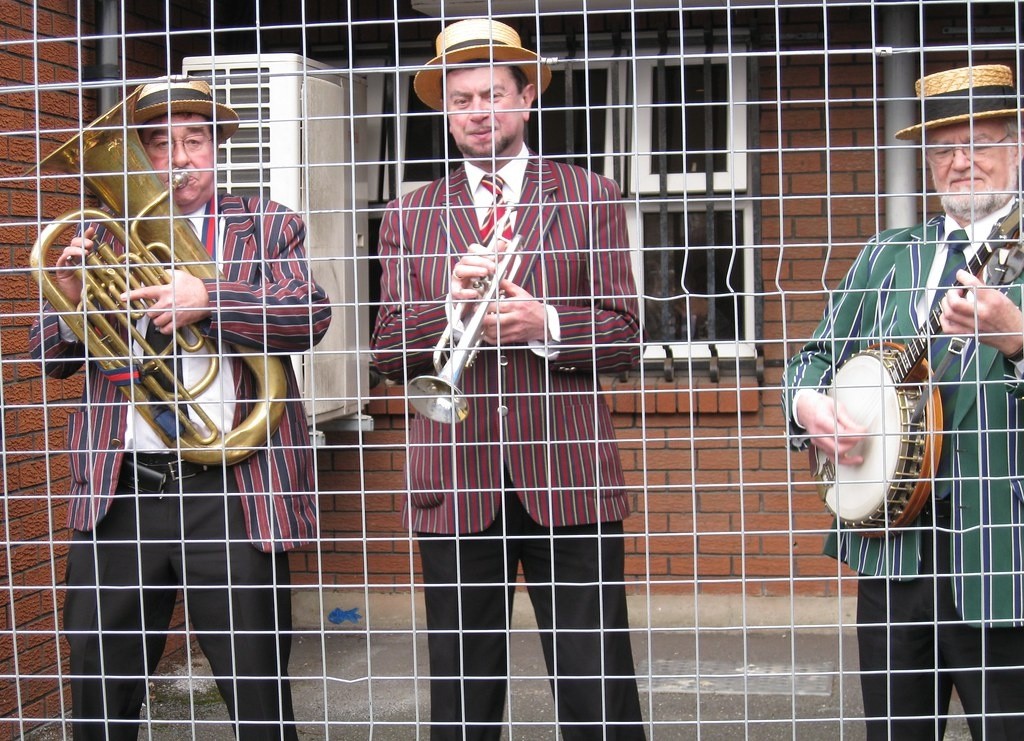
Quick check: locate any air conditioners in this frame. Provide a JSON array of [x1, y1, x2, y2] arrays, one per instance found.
[[186, 53, 373, 429]]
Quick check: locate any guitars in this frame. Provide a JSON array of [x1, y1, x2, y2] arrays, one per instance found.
[[809, 196, 1024, 537]]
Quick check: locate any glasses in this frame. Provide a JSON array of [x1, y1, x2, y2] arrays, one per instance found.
[[144, 135, 213, 151]]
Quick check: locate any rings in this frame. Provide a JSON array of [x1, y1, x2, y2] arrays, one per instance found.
[[453, 266, 464, 281]]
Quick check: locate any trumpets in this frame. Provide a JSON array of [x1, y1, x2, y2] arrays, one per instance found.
[[389, 202, 522, 424]]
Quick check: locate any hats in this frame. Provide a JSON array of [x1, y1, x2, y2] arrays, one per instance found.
[[895, 65, 1024, 140], [135, 75, 240, 142], [414, 18, 551, 112]]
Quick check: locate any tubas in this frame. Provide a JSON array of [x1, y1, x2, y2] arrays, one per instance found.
[[19, 84, 289, 466]]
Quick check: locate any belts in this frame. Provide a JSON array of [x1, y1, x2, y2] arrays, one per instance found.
[[923, 500, 951, 518], [137, 460, 208, 481]]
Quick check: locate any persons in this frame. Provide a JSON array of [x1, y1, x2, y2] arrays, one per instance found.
[[776, 60, 1023, 740], [24, 72, 335, 740], [368, 10, 654, 740]]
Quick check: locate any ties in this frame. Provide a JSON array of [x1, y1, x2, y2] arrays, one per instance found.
[[144, 318, 190, 443], [924, 230, 971, 499], [480, 175, 513, 247]]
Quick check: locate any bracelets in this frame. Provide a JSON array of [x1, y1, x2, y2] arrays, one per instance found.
[[1005, 345, 1023, 363]]
